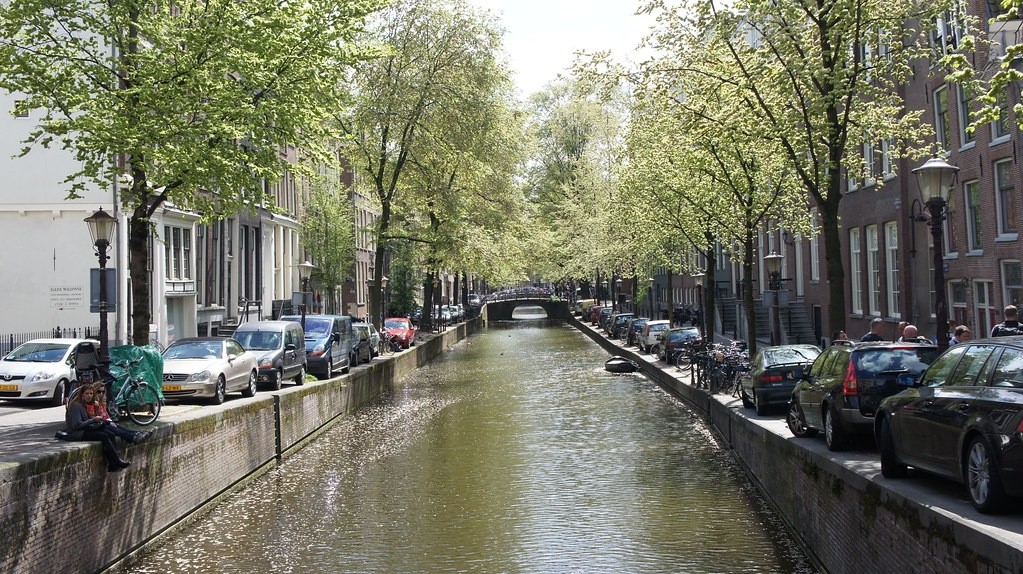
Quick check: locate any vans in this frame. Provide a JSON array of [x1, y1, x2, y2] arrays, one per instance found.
[[231, 322, 308, 390], [574, 288, 594, 301], [281, 314, 352, 379]]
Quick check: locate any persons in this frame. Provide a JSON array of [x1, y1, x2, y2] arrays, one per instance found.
[[948, 325, 969, 347], [831, 330, 848, 346], [902, 324, 925, 344], [898, 322, 910, 343], [946, 320, 955, 340], [86, 381, 153, 444], [859, 318, 884, 341], [990, 305, 1023, 337], [66, 384, 130, 472]]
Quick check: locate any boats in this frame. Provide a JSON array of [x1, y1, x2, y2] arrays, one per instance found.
[[604, 355, 640, 373]]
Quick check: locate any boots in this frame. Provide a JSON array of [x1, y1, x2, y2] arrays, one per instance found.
[[104, 442, 131, 472]]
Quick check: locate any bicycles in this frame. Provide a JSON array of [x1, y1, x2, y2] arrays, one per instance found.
[[675, 338, 751, 399], [619, 327, 628, 342], [382, 334, 401, 356], [65, 343, 162, 426], [650, 344, 658, 360]]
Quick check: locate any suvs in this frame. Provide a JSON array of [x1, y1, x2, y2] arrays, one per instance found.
[[786, 339, 937, 451]]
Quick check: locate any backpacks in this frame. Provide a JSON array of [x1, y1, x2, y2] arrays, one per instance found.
[[996, 323, 1023, 336]]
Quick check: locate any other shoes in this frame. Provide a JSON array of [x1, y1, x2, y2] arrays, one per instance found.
[[133, 431, 153, 445]]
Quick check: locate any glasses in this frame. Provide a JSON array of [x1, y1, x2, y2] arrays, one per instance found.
[[96, 390, 105, 394]]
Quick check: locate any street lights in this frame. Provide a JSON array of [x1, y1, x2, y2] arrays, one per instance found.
[[691, 270, 706, 341], [298, 260, 315, 334], [601, 280, 609, 307], [616, 278, 623, 313], [912, 157, 960, 354], [762, 250, 785, 345], [382, 276, 389, 331], [82, 207, 118, 399], [647, 277, 655, 320]]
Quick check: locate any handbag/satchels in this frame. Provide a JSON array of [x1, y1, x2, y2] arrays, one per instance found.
[[83, 417, 105, 432]]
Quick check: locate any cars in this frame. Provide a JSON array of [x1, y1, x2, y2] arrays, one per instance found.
[[736, 344, 823, 416], [160, 336, 260, 405], [657, 329, 704, 365], [411, 293, 480, 325], [607, 312, 636, 338], [575, 299, 613, 328], [874, 335, 1023, 515], [0, 338, 99, 406], [626, 318, 670, 352], [384, 318, 415, 349], [351, 323, 381, 366]]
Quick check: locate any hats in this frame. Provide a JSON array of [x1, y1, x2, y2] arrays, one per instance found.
[[1005, 305, 1018, 314]]
